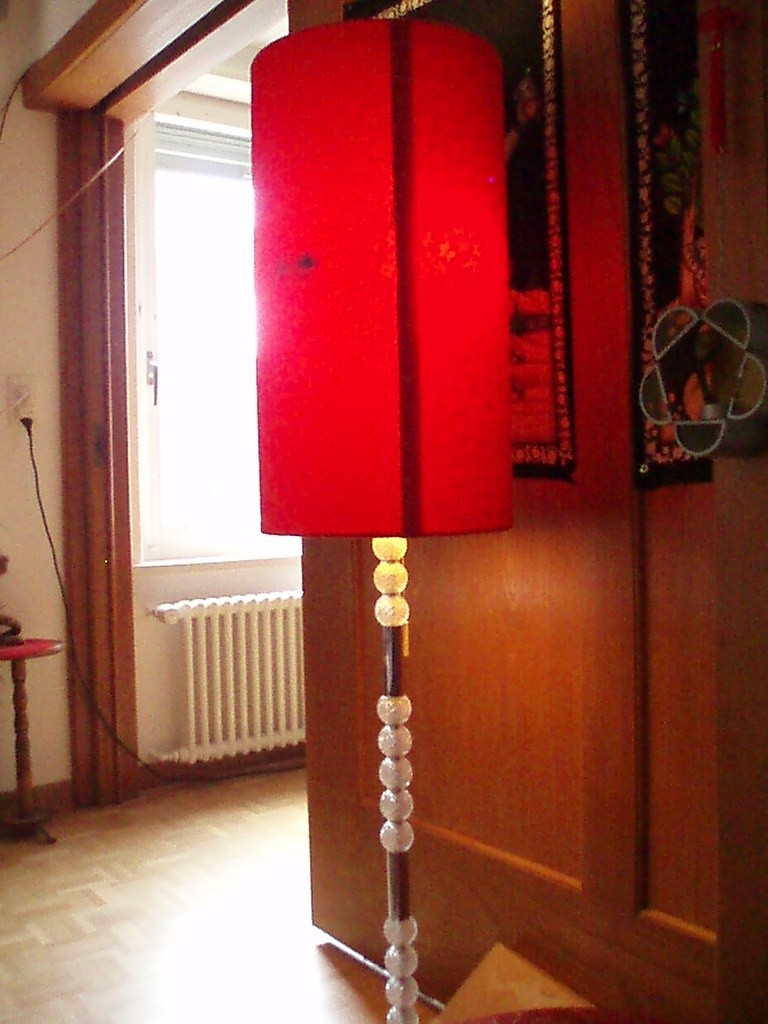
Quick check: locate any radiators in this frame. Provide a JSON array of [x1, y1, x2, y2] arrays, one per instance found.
[[147, 593, 304, 765]]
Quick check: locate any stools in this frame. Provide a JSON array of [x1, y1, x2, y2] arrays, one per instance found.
[[0, 637, 66, 841]]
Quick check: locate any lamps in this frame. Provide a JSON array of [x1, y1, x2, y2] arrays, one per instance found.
[[252, 23, 512, 1024]]
